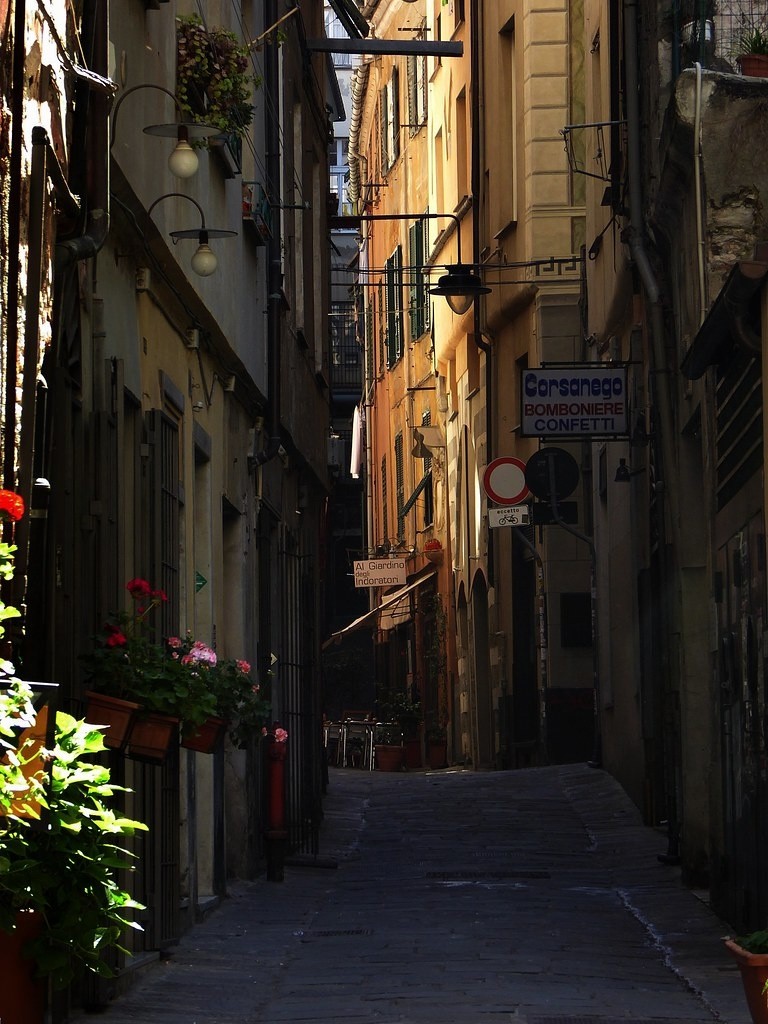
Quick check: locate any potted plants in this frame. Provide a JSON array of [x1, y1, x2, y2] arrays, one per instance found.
[[724, 929, 768, 1023], [374, 680, 424, 772], [426, 710, 447, 770]]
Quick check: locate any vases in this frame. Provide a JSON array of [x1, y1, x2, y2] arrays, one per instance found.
[[85, 689, 224, 766]]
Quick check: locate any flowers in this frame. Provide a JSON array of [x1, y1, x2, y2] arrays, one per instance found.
[[99, 577, 289, 752]]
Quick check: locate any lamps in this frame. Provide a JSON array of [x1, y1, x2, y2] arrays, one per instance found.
[[110, 84, 222, 179], [328, 214, 494, 316], [114, 191, 241, 277], [613, 457, 642, 483]]
